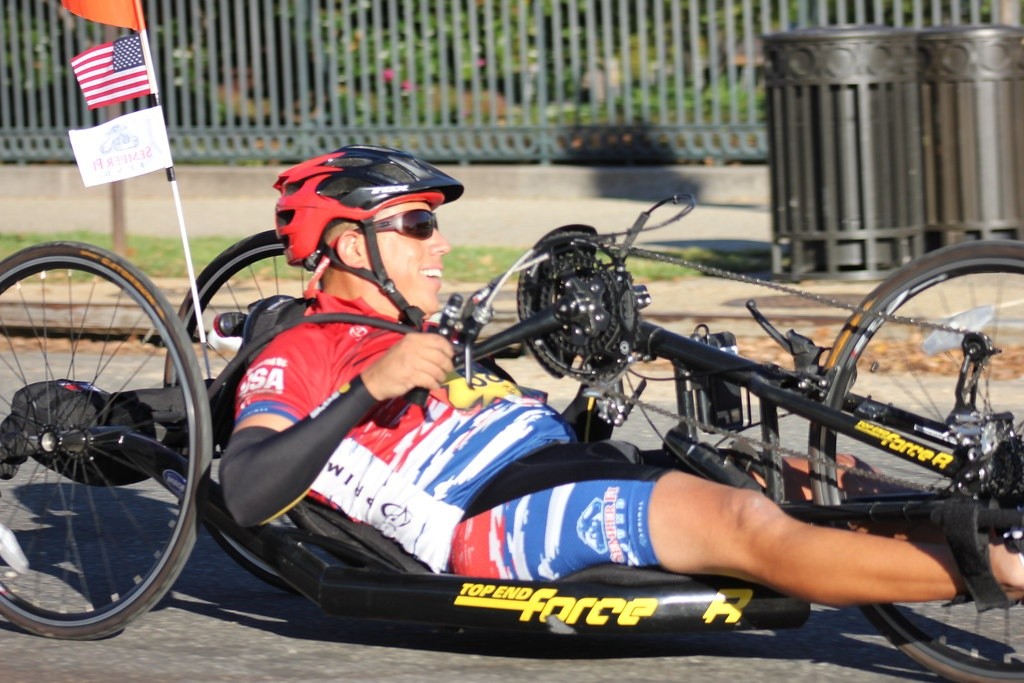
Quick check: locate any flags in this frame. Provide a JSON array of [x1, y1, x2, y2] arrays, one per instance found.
[[70, 35, 160, 109], [60, 1, 146, 33], [68, 106, 173, 188]]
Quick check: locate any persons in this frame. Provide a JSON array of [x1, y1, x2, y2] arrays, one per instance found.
[[218, 143, 1024, 604]]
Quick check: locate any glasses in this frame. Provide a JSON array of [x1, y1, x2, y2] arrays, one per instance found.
[[304, 209, 438, 299]]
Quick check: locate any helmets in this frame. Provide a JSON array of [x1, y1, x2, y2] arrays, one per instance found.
[[272, 144, 464, 271]]
